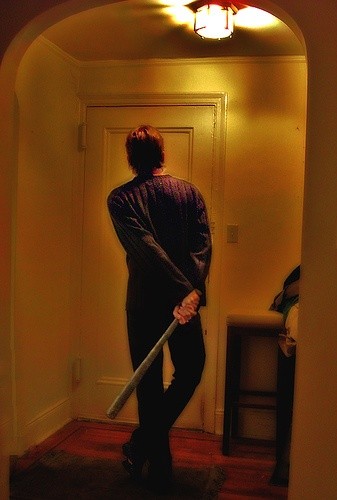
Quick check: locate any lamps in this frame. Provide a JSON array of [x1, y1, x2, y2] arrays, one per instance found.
[[183, 0, 248, 42]]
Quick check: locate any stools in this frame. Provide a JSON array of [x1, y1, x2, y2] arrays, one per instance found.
[[221, 309, 285, 454]]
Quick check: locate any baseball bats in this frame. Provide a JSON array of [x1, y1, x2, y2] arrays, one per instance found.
[[106, 288, 203, 420]]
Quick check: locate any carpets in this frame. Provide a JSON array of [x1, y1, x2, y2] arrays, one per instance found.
[[7, 448, 224, 500]]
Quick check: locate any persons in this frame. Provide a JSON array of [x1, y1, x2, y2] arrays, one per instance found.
[[106, 125, 212, 500]]
